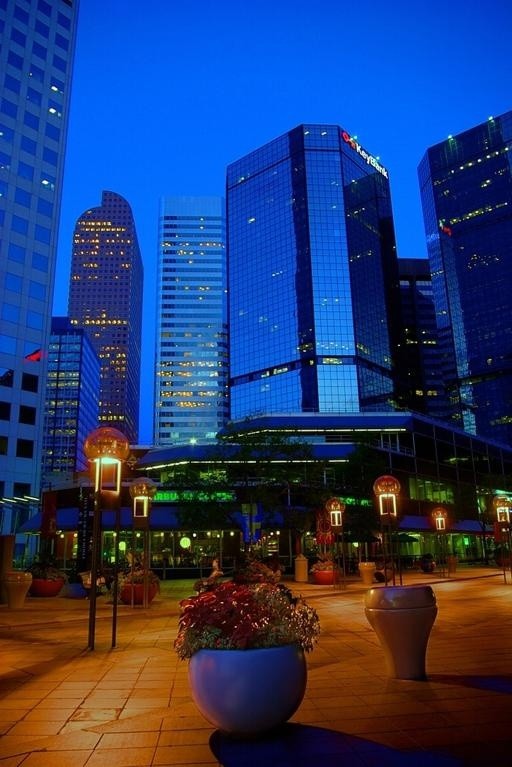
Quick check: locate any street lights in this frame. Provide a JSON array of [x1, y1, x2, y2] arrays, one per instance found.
[[431, 505, 450, 578], [374, 474, 403, 586], [82, 422, 130, 652], [492, 494, 511, 583], [325, 495, 347, 591], [129, 474, 158, 609]]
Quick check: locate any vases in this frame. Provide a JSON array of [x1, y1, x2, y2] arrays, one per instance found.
[[189, 641, 309, 732], [117, 582, 157, 604], [313, 570, 333, 584]]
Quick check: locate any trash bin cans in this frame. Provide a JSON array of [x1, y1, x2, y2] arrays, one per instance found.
[[294, 554, 308, 582]]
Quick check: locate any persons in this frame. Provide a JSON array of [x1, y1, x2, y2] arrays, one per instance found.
[[190, 558, 222, 598]]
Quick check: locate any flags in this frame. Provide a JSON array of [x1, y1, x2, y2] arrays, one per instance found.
[[24, 350, 41, 362]]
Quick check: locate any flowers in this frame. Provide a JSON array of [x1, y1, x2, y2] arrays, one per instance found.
[[308, 557, 338, 573], [123, 568, 162, 594], [173, 560, 321, 661]]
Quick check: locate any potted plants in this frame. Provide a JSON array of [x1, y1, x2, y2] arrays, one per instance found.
[[29, 565, 71, 596]]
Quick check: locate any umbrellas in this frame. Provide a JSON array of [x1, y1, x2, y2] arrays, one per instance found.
[[390, 531, 419, 545], [342, 529, 380, 564]]
